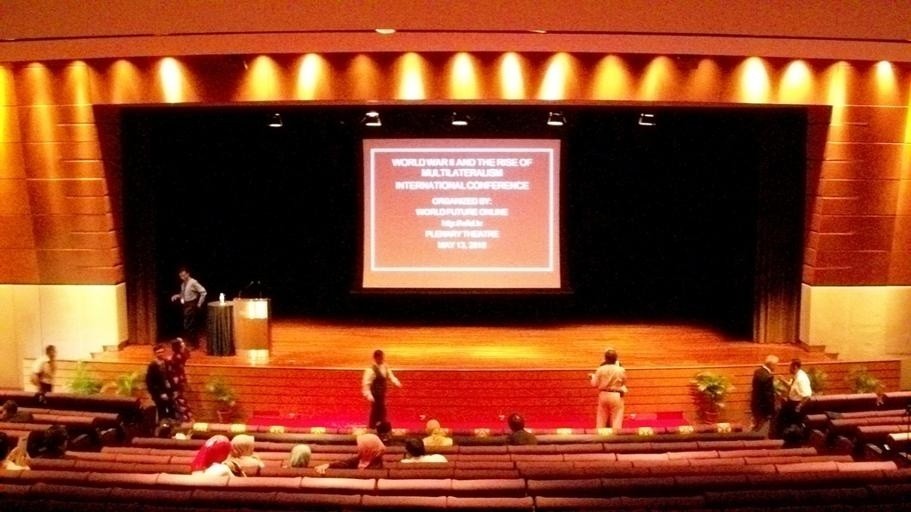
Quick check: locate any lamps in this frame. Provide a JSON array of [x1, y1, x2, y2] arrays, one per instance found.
[[547, 111, 566, 126], [638, 111, 655, 126], [451, 111, 470, 126], [365, 111, 383, 126], [268, 111, 283, 127]]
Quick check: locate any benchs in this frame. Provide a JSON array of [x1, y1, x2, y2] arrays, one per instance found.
[[0, 390, 911, 512]]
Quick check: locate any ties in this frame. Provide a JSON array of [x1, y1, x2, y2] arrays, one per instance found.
[[181, 282, 186, 304]]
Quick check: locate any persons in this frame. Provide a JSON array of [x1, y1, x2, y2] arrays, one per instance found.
[[587, 351, 626, 429], [6, 429, 48, 460], [504, 413, 536, 445], [171, 266, 207, 351], [165, 337, 193, 422], [750, 356, 780, 433], [778, 358, 812, 435], [0, 400, 35, 424], [358, 349, 402, 429], [1, 430, 29, 470], [41, 425, 71, 458], [32, 344, 54, 394], [190, 420, 455, 481], [154, 423, 174, 441], [144, 345, 177, 421]]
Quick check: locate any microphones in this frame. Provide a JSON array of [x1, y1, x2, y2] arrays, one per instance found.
[[257, 280, 263, 299], [236, 281, 255, 299]]
